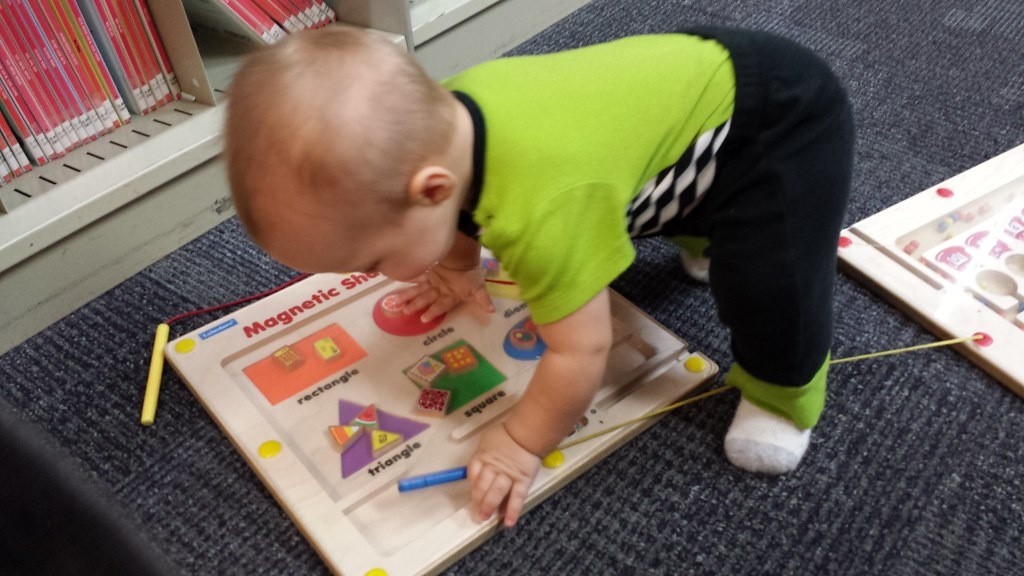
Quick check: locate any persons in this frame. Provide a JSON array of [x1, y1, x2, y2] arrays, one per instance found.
[[222, 18, 860, 528]]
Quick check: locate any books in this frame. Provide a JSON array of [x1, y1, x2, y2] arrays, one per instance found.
[[183, 0, 345, 46], [0, 0, 184, 186]]
